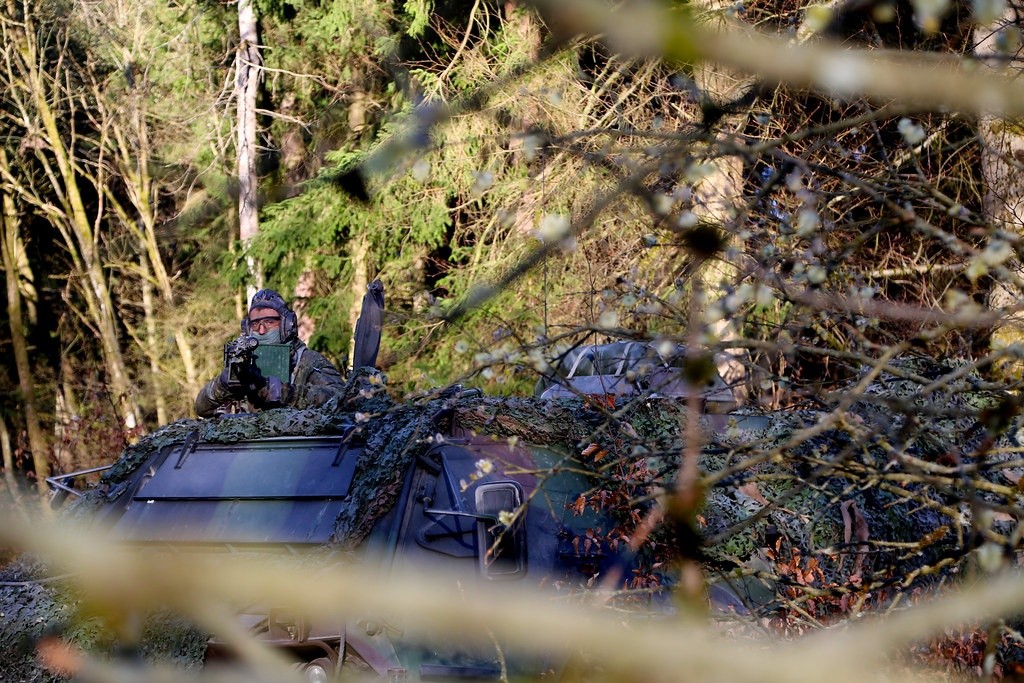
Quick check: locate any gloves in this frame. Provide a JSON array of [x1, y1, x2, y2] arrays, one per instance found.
[[233, 363, 266, 390]]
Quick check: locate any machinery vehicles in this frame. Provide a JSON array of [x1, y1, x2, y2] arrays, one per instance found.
[[1, 276, 1024, 683]]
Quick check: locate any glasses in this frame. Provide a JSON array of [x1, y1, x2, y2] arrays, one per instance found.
[[248, 316, 280, 331]]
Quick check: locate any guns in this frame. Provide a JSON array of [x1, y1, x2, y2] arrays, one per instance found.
[[224, 337, 260, 380]]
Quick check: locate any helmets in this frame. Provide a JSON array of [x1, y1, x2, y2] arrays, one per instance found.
[[241, 289, 298, 343]]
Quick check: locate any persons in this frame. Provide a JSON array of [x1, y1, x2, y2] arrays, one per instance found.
[[194, 289, 345, 417]]
